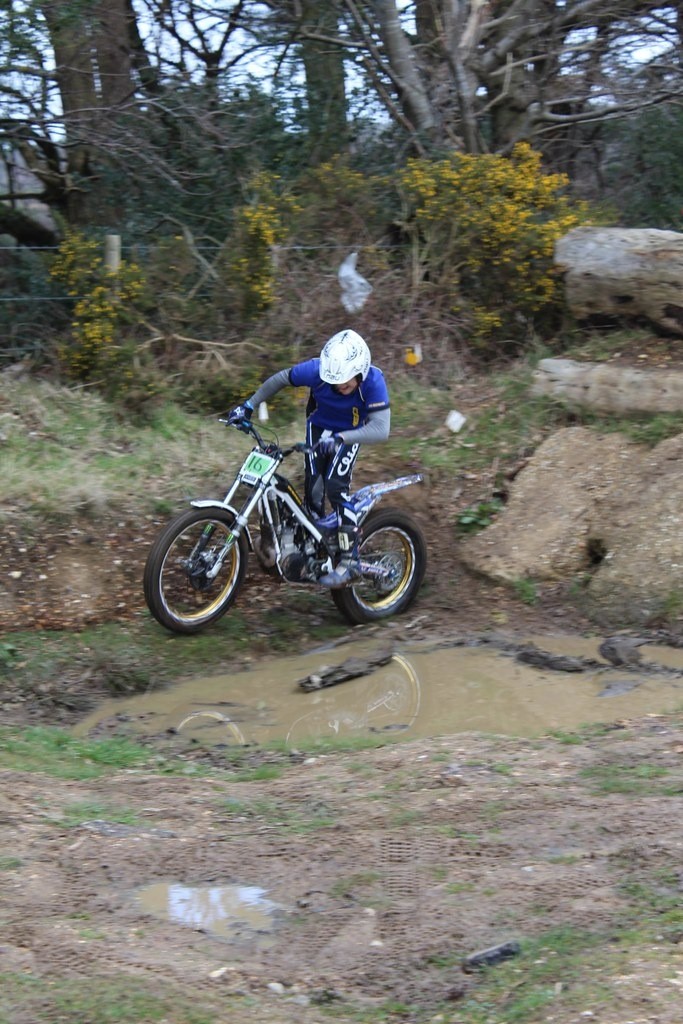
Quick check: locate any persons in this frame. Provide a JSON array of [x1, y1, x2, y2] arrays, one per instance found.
[[224, 329, 391, 590]]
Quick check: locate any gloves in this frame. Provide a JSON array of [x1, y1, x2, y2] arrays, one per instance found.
[[308, 432, 344, 459], [225, 401, 254, 435]]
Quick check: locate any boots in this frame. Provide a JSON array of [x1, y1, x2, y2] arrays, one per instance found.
[[318, 525, 362, 588]]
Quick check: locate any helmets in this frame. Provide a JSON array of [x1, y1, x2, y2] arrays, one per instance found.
[[319, 329, 371, 384]]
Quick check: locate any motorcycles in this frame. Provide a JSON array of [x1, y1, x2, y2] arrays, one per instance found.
[[172, 654, 422, 753], [143, 416, 427, 634]]
